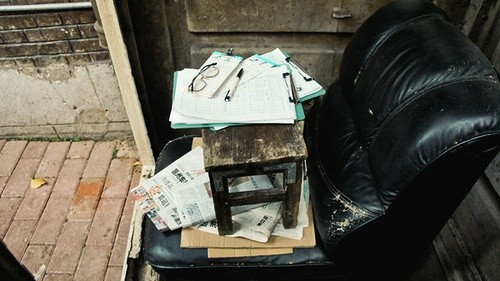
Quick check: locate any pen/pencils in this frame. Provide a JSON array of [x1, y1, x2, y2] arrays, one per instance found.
[[224, 68, 243, 102]]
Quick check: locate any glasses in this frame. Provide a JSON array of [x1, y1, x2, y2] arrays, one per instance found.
[[187, 62, 219, 92]]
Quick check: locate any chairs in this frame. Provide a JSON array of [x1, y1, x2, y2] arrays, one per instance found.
[[144, 0, 500, 281]]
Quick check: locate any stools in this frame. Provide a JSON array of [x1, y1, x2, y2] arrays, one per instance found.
[[201, 122, 308, 236]]
